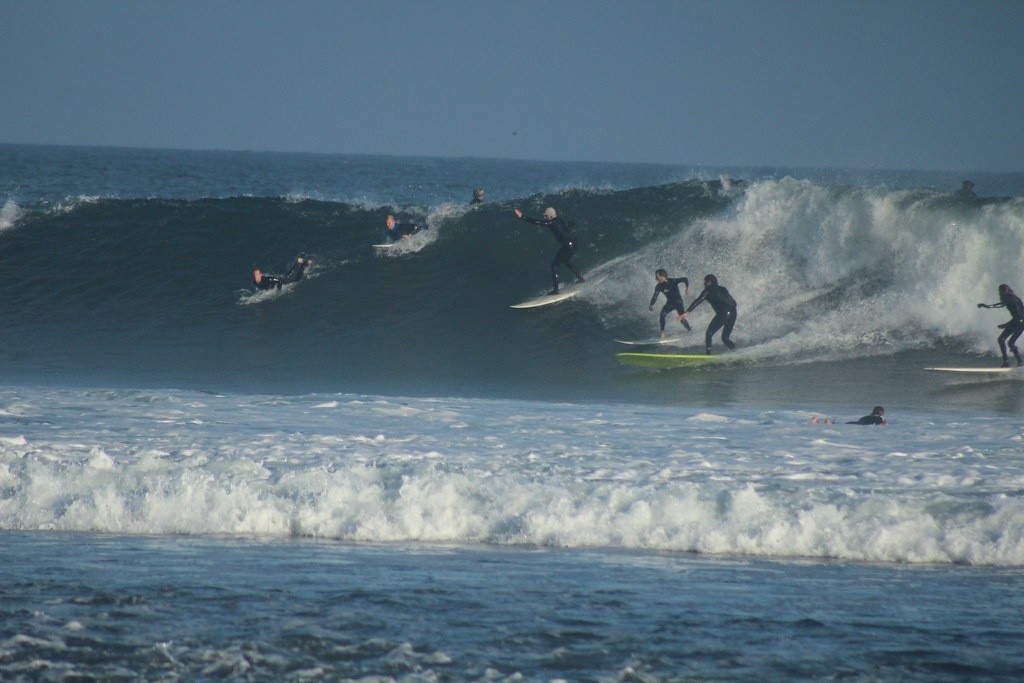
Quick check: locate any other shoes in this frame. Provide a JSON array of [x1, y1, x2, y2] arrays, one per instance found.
[[546, 290, 559, 295], [575, 279, 584, 283], [1000, 362, 1009, 367], [1016, 361, 1023, 366]]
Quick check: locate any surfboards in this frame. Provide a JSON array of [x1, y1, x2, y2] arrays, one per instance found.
[[614, 338, 681, 345], [923, 365, 1021, 372], [372, 243, 395, 249], [615, 353, 718, 367], [509, 272, 609, 308]]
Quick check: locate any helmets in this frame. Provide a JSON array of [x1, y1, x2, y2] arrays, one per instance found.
[[542, 207, 555, 219]]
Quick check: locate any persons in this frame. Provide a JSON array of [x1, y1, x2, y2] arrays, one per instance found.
[[809, 406, 886, 425], [678, 275, 738, 355], [649, 269, 691, 338], [515, 208, 585, 296], [471, 188, 484, 205], [386, 216, 419, 240], [978, 284, 1024, 367], [954, 180, 976, 197], [252, 258, 313, 290]]
